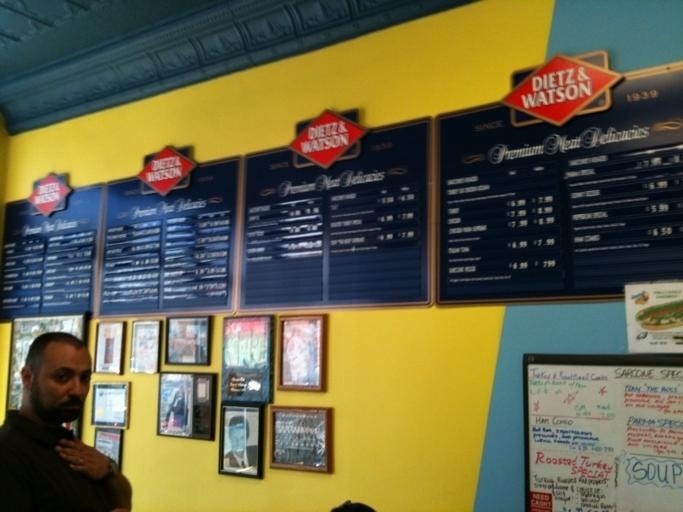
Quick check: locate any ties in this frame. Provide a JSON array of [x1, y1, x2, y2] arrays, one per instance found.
[[242, 461, 246, 468]]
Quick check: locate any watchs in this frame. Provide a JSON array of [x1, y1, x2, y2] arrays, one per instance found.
[[100, 456, 118, 485]]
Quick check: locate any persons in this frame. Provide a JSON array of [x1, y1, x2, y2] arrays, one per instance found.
[[287, 328, 310, 384], [162, 384, 185, 428], [225, 417, 258, 467], [0, 332, 131, 510]]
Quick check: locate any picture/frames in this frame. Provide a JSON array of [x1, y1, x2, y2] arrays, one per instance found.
[[129, 320, 163, 373], [165, 315, 212, 366], [218, 401, 264, 480], [269, 405, 333, 474], [91, 381, 131, 430], [156, 370, 217, 441], [94, 427, 124, 472], [93, 320, 127, 376], [277, 313, 328, 393], [220, 314, 276, 404]]
